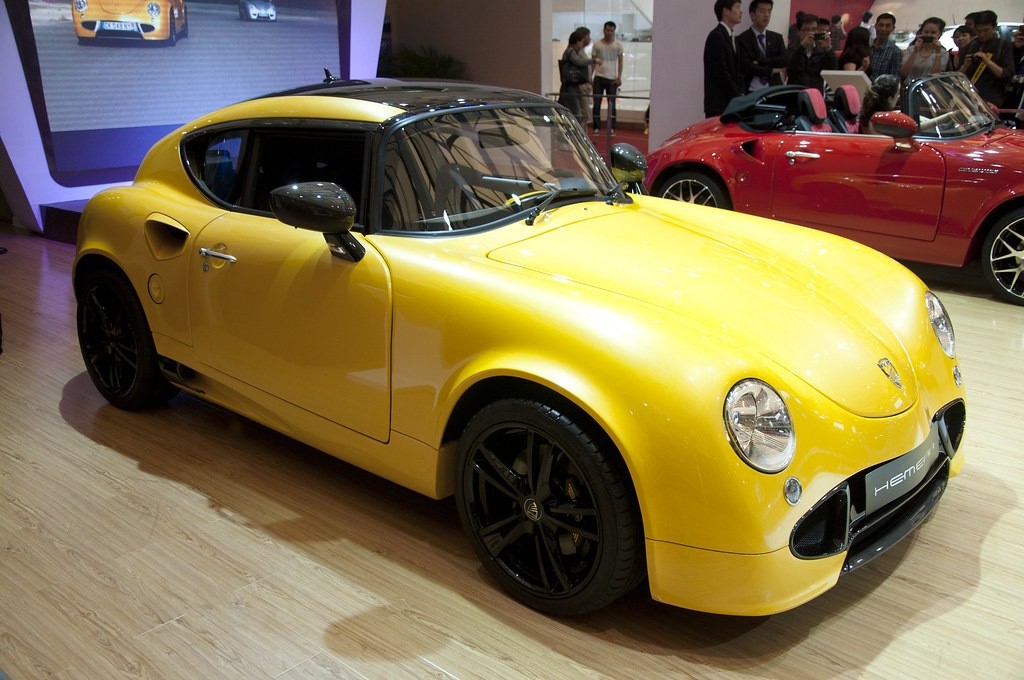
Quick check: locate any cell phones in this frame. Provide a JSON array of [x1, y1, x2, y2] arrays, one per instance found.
[[814, 33, 825, 40], [920, 36, 934, 43]]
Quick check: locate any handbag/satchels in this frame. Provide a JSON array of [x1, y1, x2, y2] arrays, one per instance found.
[[559, 46, 589, 85]]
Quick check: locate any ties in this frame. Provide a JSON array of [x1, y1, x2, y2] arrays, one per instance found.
[[758, 34, 766, 53], [730, 35, 733, 41]]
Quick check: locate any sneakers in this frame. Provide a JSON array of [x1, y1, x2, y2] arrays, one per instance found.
[[593, 128, 599, 136], [611, 129, 616, 137]]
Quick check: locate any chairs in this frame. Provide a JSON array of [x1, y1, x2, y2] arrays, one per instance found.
[[796, 87, 840, 134], [828, 84, 863, 134]]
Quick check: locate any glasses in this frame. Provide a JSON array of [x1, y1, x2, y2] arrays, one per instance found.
[[799, 27, 818, 33]]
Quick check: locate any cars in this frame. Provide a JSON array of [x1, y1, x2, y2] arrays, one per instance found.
[[70, 76, 967, 617], [71, 0, 189, 46], [239, 0, 277, 22], [644, 72, 1024, 302], [896, 23, 1024, 51]]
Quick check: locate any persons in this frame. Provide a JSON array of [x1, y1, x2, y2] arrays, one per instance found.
[[558, 21, 602, 137], [644, 105, 650, 134], [591, 22, 623, 136], [736, 0, 787, 95], [859, 74, 970, 133], [703, 0, 745, 119], [786, 10, 1024, 132]]
